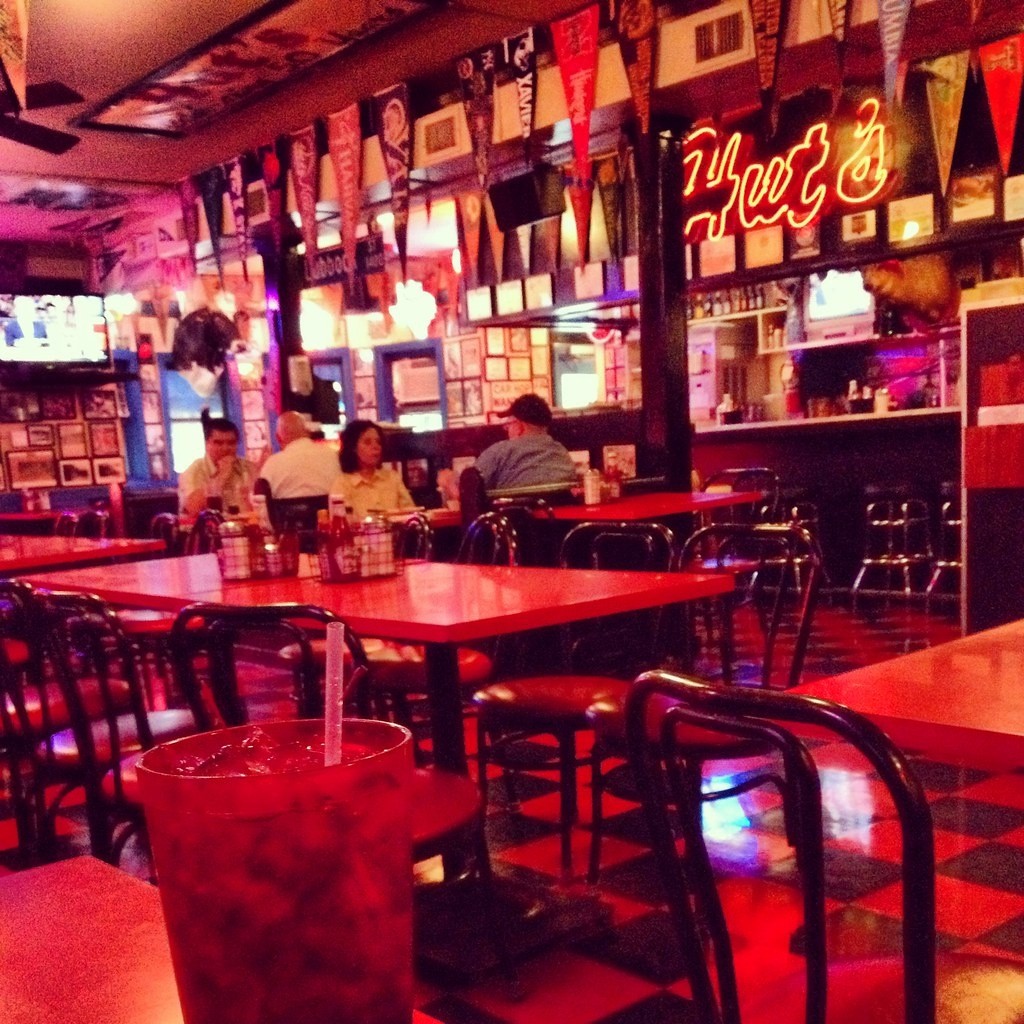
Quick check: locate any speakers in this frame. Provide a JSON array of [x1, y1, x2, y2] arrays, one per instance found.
[[489, 170, 566, 230]]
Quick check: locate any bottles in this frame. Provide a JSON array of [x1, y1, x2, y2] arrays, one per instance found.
[[248, 494, 275, 577], [205, 495, 224, 551], [345, 506, 356, 528], [316, 510, 334, 583], [227, 505, 239, 522], [218, 521, 246, 540], [331, 499, 360, 582], [922, 374, 938, 407], [715, 393, 734, 424], [606, 452, 621, 500]]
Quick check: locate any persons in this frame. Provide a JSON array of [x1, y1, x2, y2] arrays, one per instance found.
[[253, 411, 343, 553], [449, 393, 577, 511], [177, 419, 258, 526], [3, 296, 48, 347], [328, 419, 419, 521]]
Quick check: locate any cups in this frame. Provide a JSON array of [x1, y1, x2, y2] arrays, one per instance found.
[[763, 392, 787, 421], [217, 538, 249, 581], [584, 470, 600, 504], [136, 718, 413, 1024]]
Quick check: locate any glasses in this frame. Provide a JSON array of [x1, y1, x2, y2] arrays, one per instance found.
[[501, 420, 518, 430]]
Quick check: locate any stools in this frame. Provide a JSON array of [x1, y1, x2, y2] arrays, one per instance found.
[[474, 674, 630, 870], [924, 480, 961, 617], [366, 644, 492, 768], [752, 486, 832, 608], [586, 689, 801, 944], [278, 638, 398, 718], [851, 483, 933, 617]]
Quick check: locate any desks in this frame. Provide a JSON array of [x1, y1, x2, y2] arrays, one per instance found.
[[14, 552, 425, 725], [0, 508, 108, 534], [179, 518, 259, 537], [178, 562, 734, 986], [0, 534, 167, 684], [421, 507, 461, 529], [526, 491, 764, 671], [0, 857, 446, 1024], [770, 618, 1024, 766]]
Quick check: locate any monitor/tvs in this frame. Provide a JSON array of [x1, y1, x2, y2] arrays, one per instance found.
[[0, 293, 110, 369]]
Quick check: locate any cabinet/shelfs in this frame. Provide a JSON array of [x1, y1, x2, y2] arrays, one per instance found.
[[686, 304, 878, 356]]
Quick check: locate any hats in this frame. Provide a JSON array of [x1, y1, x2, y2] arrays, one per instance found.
[[496, 393, 552, 427]]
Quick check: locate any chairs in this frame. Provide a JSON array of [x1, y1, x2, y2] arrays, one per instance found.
[[0, 467, 934, 1024]]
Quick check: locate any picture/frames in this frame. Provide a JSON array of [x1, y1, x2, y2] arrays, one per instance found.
[[442, 307, 550, 429], [0, 388, 126, 493]]
